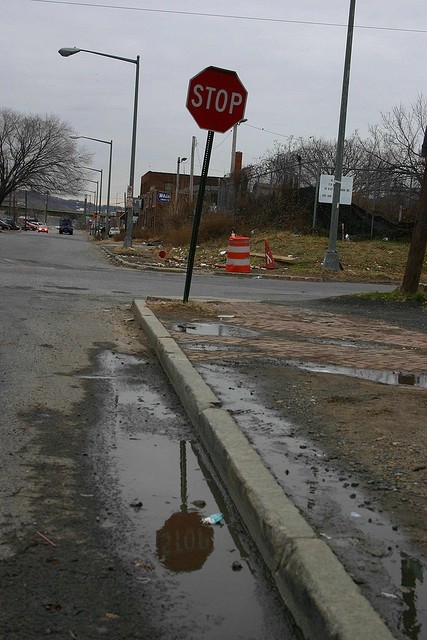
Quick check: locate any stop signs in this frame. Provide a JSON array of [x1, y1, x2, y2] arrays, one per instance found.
[[184, 66, 248, 134], [155, 435, 216, 573]]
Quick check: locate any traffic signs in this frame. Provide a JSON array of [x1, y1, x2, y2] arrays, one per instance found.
[[319, 175, 353, 207]]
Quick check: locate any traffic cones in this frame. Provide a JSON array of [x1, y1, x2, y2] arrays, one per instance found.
[[264, 241, 276, 269], [225, 235, 251, 272]]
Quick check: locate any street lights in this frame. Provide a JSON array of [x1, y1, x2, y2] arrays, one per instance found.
[[71, 177, 97, 237], [70, 135, 113, 241], [73, 165, 102, 238], [74, 188, 96, 235], [58, 47, 140, 248]]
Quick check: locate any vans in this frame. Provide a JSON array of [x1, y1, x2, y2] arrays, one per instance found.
[[59, 215, 73, 235]]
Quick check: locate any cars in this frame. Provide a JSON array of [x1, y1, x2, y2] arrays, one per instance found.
[[108, 227, 122, 236], [1, 215, 41, 231], [38, 225, 48, 233]]
